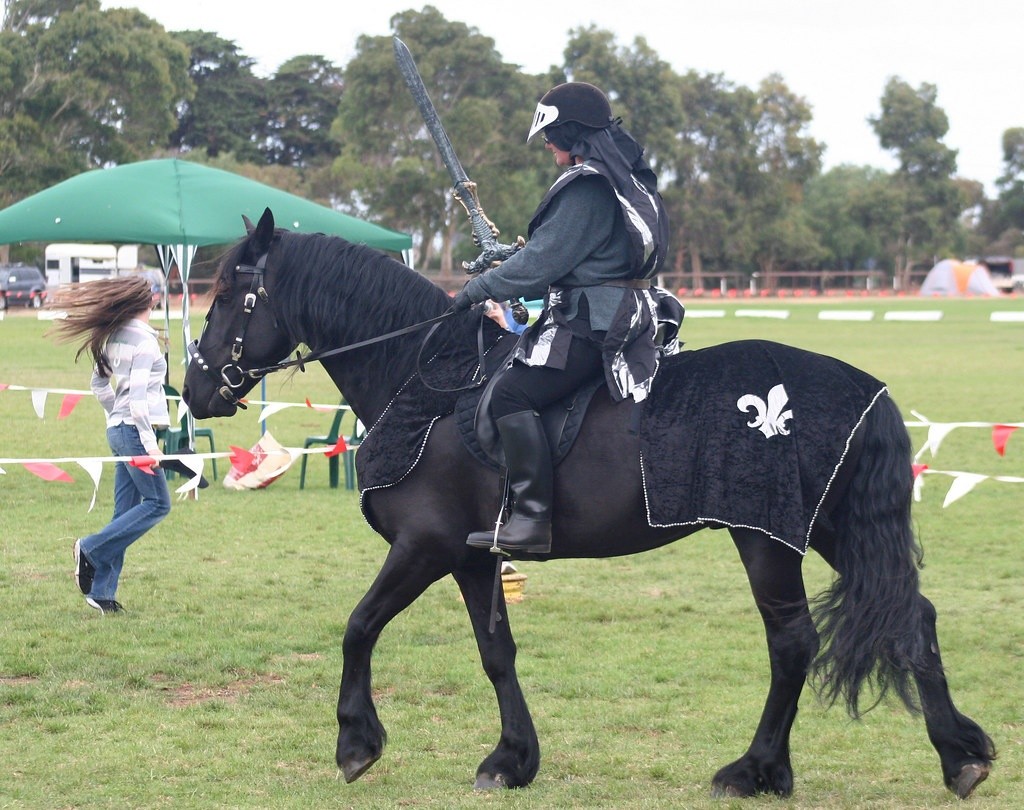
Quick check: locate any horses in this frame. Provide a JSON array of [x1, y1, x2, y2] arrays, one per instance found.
[[182, 207, 999, 799]]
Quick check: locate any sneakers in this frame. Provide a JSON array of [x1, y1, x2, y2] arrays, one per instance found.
[[86, 598, 126, 617], [73, 539, 96, 595]]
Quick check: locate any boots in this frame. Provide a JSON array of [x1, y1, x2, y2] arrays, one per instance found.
[[467, 410, 554, 553]]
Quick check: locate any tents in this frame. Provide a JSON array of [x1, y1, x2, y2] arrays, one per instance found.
[[0, 160, 411, 252]]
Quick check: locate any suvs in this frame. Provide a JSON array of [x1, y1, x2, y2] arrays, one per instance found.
[[0, 263, 49, 313]]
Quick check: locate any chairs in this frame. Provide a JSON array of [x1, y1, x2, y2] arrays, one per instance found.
[[299, 399, 361, 494], [152, 384, 217, 480]]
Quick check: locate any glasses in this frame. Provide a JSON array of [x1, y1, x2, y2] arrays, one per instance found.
[[541, 137, 550, 144]]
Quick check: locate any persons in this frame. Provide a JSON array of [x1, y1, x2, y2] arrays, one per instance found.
[[43, 273, 174, 616], [454, 83, 685, 554]]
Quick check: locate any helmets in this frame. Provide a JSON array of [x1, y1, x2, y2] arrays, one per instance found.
[[525, 82, 613, 144]]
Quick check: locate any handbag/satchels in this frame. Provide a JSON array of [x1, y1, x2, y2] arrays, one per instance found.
[[223, 431, 292, 491]]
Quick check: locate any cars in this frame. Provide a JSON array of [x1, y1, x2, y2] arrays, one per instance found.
[[137, 270, 162, 311]]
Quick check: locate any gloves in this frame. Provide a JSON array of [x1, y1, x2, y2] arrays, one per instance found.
[[451, 283, 472, 313]]
